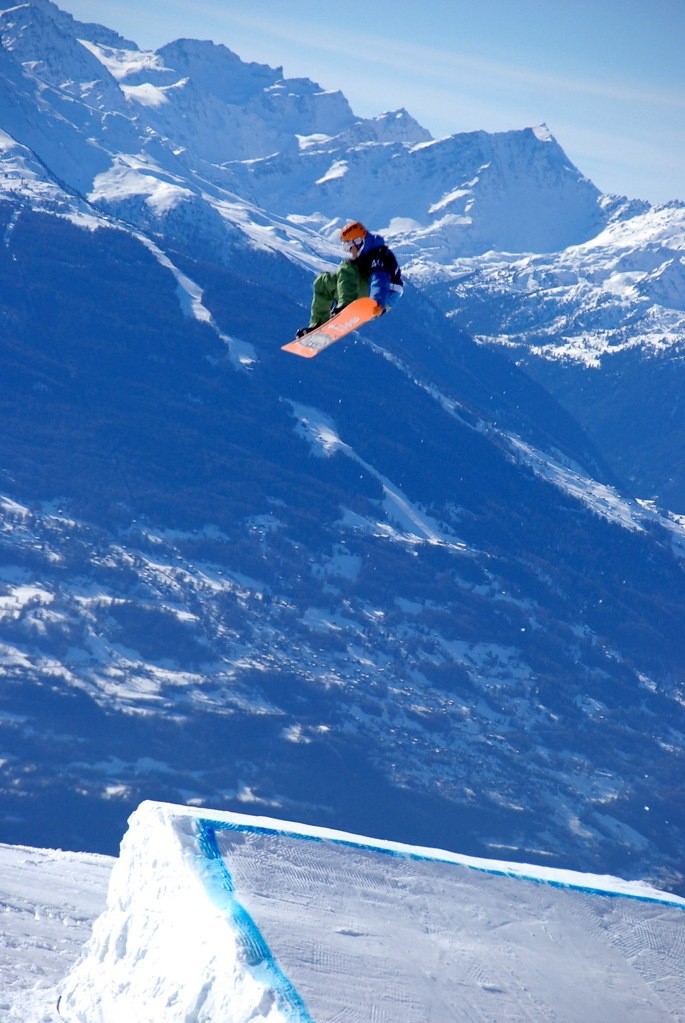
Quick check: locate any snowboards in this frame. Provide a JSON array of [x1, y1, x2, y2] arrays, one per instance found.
[[279, 295, 380, 360]]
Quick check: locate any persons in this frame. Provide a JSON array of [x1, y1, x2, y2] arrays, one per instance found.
[[295, 222, 404, 341]]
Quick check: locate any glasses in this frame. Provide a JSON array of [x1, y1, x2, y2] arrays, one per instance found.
[[342, 242, 351, 252]]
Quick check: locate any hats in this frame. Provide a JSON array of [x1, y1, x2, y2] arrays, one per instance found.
[[339, 221, 367, 244]]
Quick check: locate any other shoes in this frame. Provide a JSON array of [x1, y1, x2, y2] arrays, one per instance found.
[[330, 305, 346, 317], [295, 323, 320, 338]]
